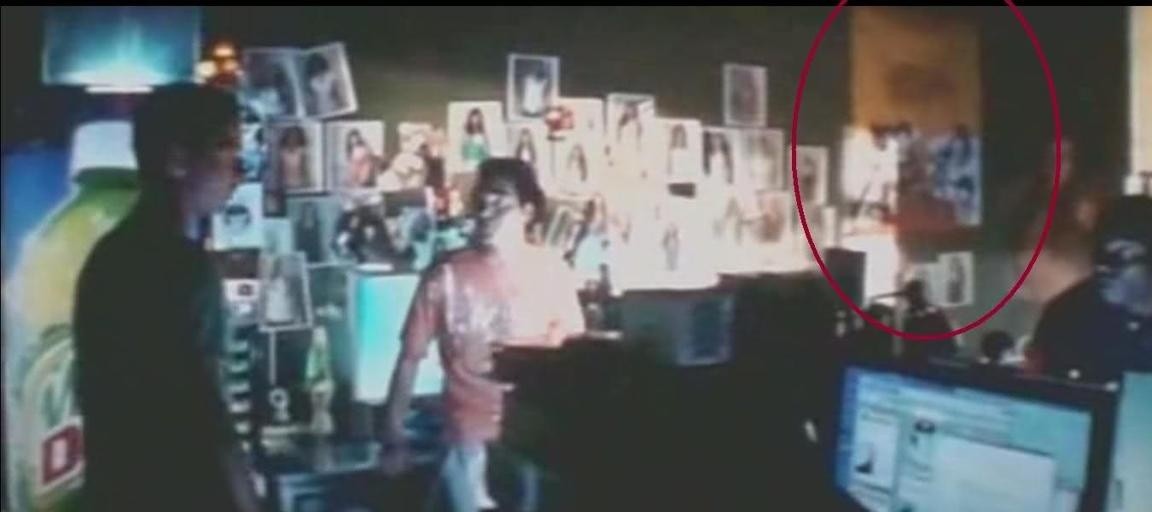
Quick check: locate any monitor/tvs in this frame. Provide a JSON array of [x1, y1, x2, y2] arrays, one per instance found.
[[830, 356, 1120, 512]]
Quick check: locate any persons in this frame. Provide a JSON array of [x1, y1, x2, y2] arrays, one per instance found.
[[75, 84, 263, 512], [224, 54, 977, 328], [379, 159, 586, 512], [1031, 195, 1151, 388], [983, 330, 1043, 377]]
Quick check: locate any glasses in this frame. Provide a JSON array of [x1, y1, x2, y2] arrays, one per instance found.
[[1096, 248, 1147, 270]]
